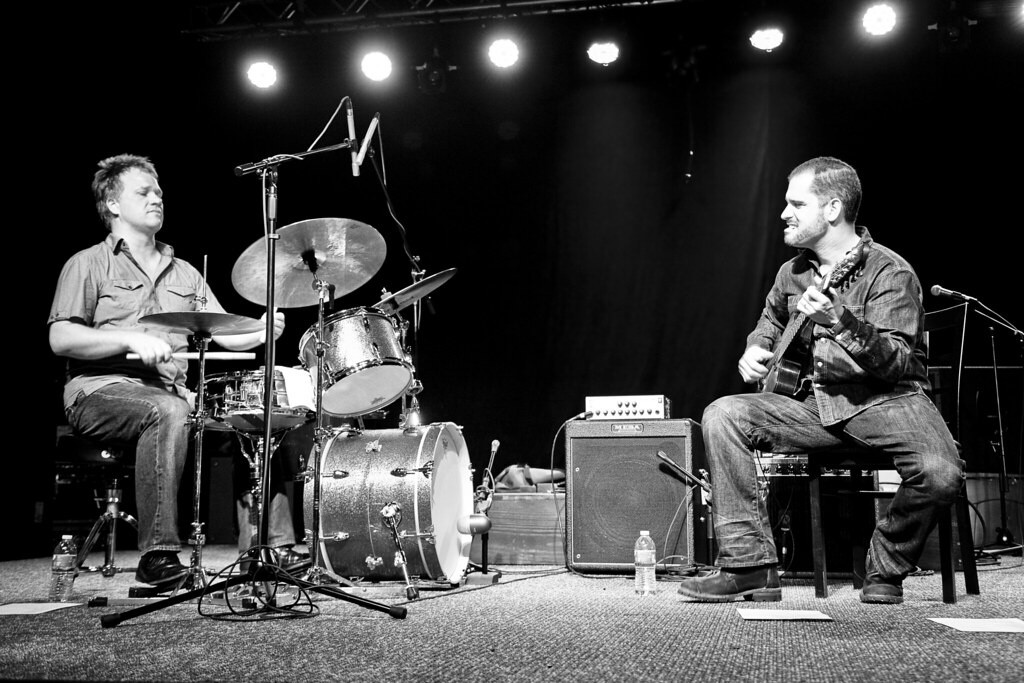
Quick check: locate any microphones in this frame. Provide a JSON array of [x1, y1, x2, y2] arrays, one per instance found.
[[931, 285, 978, 302], [346, 96, 360, 177], [457, 514, 493, 535], [355, 112, 381, 166]]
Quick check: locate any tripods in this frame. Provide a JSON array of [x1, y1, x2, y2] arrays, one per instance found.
[[99, 139, 407, 635], [298, 268, 359, 588]]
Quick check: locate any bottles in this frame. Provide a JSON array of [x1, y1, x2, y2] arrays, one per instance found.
[[633, 530, 656, 596], [47, 534, 78, 604]]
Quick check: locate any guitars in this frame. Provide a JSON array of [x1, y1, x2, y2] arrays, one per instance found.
[[762, 239, 872, 395]]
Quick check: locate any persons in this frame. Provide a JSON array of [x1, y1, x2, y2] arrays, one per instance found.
[[48, 153, 311, 585], [677, 158, 965, 604]]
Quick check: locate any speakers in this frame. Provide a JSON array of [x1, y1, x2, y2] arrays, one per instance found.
[[565, 419, 712, 571], [754, 457, 879, 577]]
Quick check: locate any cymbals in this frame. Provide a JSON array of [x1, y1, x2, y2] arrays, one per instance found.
[[372, 265, 459, 316], [230, 216, 388, 309], [138, 310, 268, 337]]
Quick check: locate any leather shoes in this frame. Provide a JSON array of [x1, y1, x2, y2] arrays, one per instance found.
[[242, 542, 311, 572], [678, 561, 783, 603], [135, 548, 196, 589], [860, 550, 904, 603]]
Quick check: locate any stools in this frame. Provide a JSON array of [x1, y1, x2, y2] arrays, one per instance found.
[[65, 444, 148, 576], [807, 437, 979, 604]]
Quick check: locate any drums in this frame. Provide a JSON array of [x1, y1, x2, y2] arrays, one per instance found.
[[302, 421, 475, 586], [297, 305, 414, 419], [197, 370, 308, 432]]
